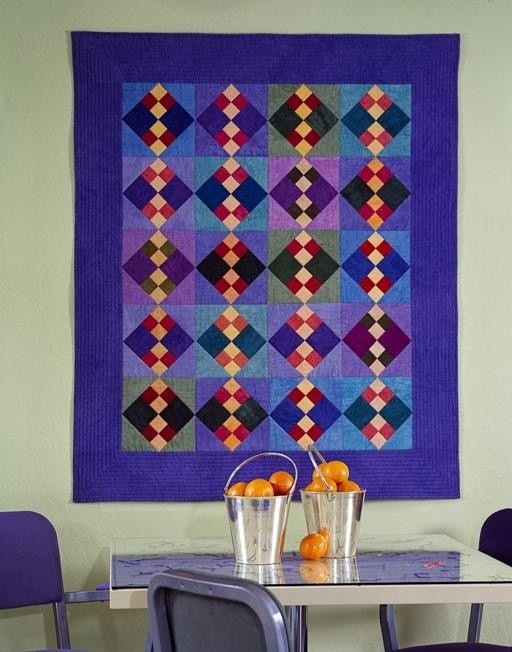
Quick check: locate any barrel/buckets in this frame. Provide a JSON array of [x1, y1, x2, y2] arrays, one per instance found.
[[222, 453, 298, 565], [233, 563, 285, 586], [320, 556, 361, 585], [298, 444, 367, 560]]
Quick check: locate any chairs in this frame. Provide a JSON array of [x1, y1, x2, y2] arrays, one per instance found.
[[146, 565, 293, 652], [0, 510, 108, 652], [386, 508, 512, 652]]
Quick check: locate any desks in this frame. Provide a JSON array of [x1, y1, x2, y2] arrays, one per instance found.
[[107, 538, 512, 652]]
